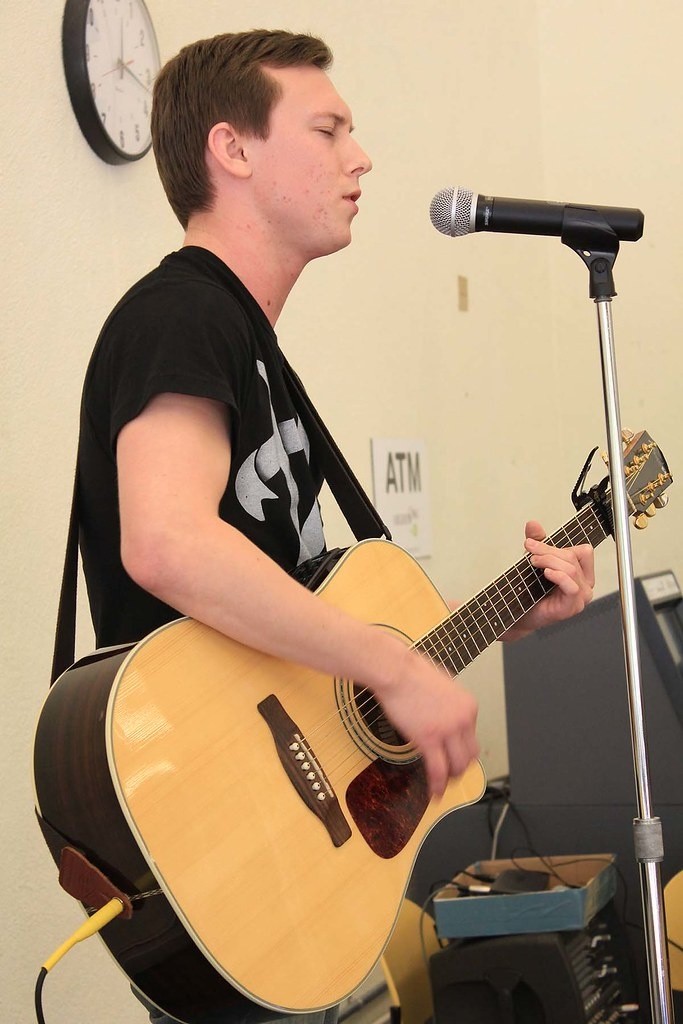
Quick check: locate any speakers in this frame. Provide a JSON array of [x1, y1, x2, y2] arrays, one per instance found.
[[427, 912, 629, 1024]]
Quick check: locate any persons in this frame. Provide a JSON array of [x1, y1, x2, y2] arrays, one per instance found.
[[74, 29, 595, 1024]]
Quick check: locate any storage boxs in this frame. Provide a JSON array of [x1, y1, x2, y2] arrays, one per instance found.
[[434, 854, 618, 937]]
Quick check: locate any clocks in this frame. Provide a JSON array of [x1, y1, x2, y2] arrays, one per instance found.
[[63, 1, 164, 165]]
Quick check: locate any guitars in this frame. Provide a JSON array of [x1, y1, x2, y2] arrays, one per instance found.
[[33, 427, 675, 1024]]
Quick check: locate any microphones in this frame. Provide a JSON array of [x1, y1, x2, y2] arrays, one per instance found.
[[429, 186, 644, 242]]
